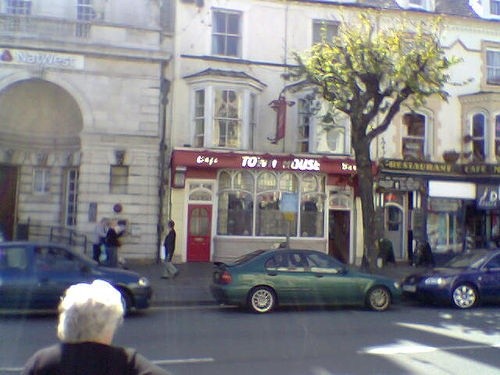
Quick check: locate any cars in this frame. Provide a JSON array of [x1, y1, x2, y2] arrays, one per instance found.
[[210, 248, 402, 315], [0, 240, 153, 318], [402, 248, 500, 310]]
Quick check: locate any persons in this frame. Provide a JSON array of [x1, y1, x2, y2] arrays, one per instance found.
[[21, 280, 170, 374], [160, 220, 181, 279], [89, 216, 126, 267]]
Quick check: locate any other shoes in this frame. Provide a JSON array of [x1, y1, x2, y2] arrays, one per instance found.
[[161, 276, 169, 280], [174, 272, 178, 279]]
